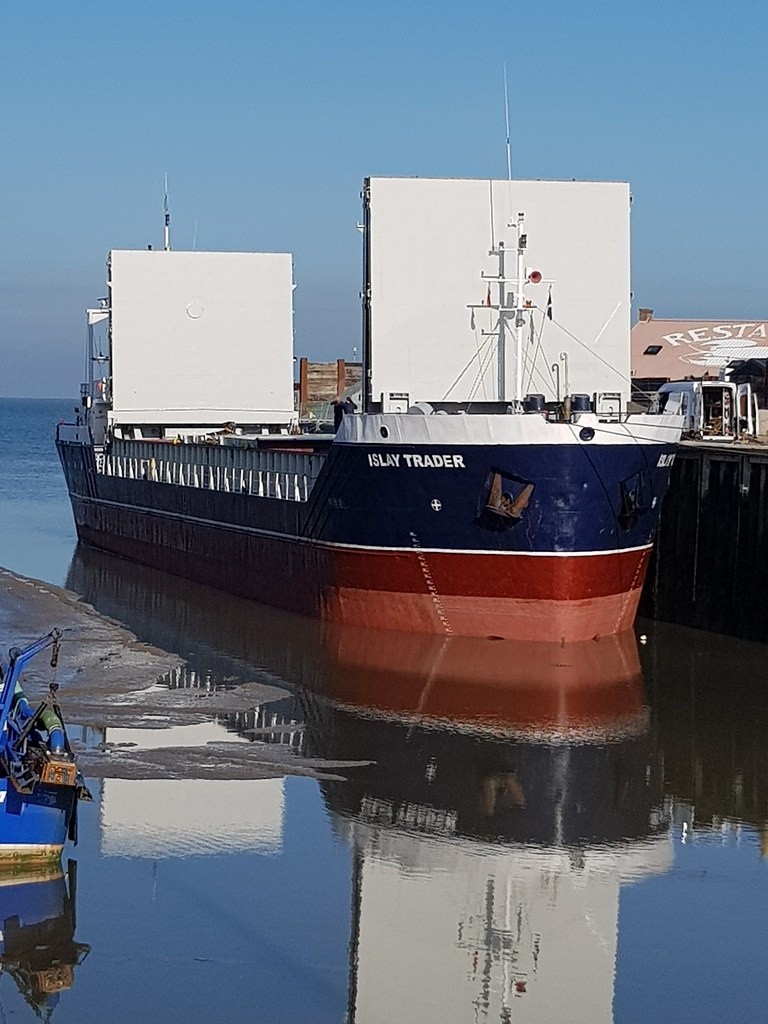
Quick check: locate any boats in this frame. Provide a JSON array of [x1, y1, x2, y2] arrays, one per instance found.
[[46, 543, 670, 889], [0, 627, 102, 896], [56, 125, 688, 643], [0, 855, 93, 1024]]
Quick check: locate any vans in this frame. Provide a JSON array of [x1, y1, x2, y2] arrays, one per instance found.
[[648, 379, 761, 443]]
